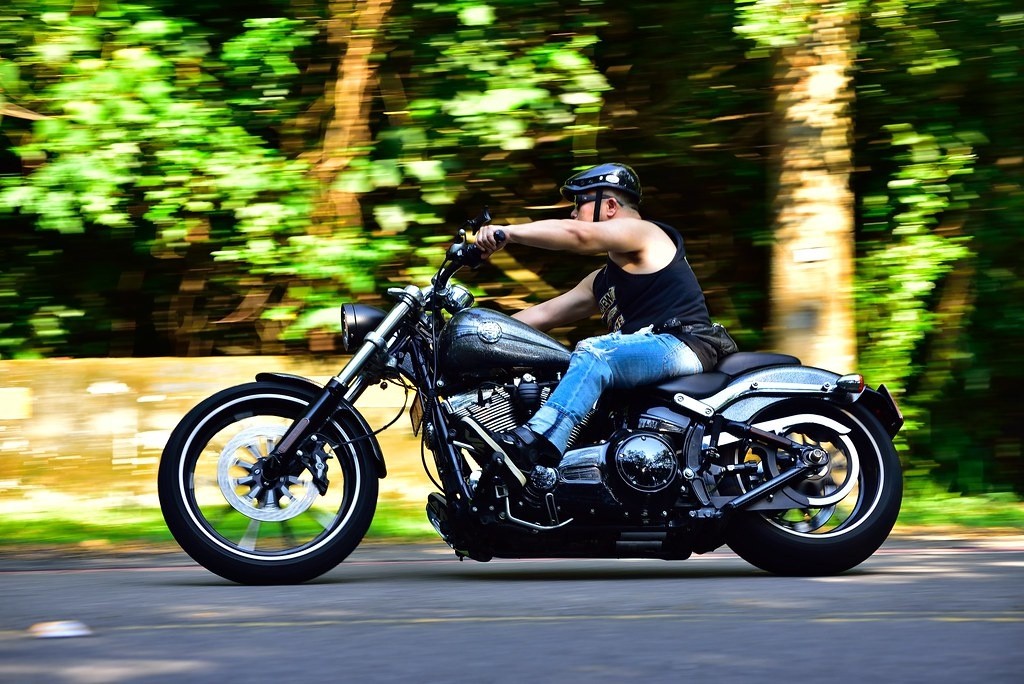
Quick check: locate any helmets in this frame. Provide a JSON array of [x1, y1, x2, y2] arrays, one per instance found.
[[560, 162, 643, 205]]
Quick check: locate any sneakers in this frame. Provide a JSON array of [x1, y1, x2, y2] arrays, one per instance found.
[[461, 415, 541, 490]]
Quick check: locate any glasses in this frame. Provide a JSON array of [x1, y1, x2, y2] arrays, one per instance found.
[[574, 194, 625, 211]]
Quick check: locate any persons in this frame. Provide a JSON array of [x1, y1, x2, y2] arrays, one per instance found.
[[458, 163, 717, 490]]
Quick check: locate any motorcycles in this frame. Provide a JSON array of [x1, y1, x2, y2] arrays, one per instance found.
[[157, 211, 904, 586]]
